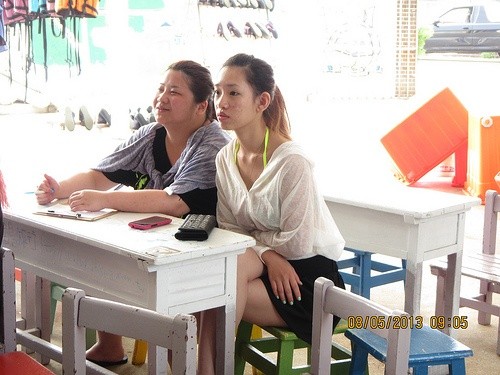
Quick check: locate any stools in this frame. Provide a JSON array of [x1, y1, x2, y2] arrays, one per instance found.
[[345, 324, 474, 375], [235, 319, 370, 375], [337, 248, 407, 300]]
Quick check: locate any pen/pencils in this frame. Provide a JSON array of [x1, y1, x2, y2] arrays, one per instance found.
[[24, 189, 54, 194]]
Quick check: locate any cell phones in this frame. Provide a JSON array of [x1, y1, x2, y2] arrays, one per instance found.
[[128, 216, 172, 230]]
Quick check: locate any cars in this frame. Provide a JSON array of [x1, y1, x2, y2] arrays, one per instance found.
[[421, 2, 500, 56]]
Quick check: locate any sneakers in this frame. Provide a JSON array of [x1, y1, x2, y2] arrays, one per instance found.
[[198, 0, 278, 42]]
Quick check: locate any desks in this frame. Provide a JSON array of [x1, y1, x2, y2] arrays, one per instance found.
[[0, 193, 256, 375], [322, 185, 481, 340]]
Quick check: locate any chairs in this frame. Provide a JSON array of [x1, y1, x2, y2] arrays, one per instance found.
[[310, 277, 413, 375], [430, 190, 500, 357], [62, 287, 198, 375]]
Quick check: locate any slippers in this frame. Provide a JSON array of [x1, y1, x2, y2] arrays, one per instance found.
[[86, 354, 128, 367]]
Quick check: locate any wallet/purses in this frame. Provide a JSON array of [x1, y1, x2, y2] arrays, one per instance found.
[[175, 214, 216, 241]]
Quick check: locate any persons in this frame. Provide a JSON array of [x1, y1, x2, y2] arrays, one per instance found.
[[194, 53, 346, 375], [35, 60, 232, 365]]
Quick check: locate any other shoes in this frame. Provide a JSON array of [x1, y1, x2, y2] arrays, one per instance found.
[[98, 105, 157, 130], [79, 106, 94, 130], [64, 106, 75, 131]]
